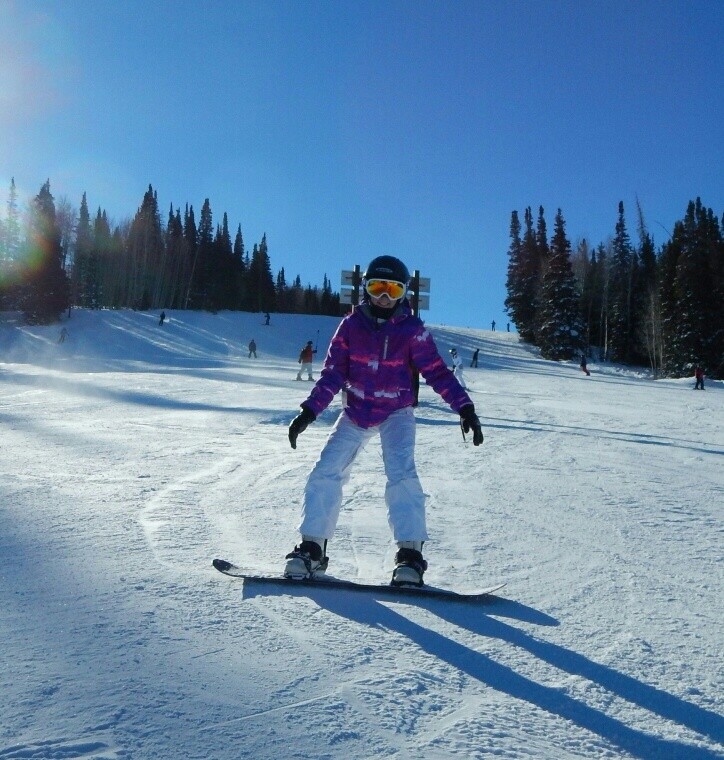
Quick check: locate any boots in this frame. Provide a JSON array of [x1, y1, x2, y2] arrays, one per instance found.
[[392, 541, 424, 585], [286, 537, 328, 578]]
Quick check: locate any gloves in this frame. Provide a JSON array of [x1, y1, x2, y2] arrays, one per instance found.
[[289, 408, 313, 449], [459, 404, 483, 447]]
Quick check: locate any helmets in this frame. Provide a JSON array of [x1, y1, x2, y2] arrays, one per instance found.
[[449, 348, 456, 354], [366, 254, 408, 284]]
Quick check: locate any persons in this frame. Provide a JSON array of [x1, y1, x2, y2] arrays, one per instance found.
[[580, 355, 590, 376], [57, 326, 70, 344], [450, 349, 466, 390], [264, 312, 270, 325], [694, 363, 705, 390], [284, 255, 484, 587], [295, 341, 317, 381], [470, 349, 480, 368], [491, 320, 496, 331], [159, 311, 165, 326], [248, 339, 257, 358], [507, 322, 510, 332]]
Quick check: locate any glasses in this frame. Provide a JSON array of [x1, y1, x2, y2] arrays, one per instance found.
[[365, 278, 405, 300]]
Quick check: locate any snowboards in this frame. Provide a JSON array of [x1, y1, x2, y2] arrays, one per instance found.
[[212, 559, 507, 596]]
